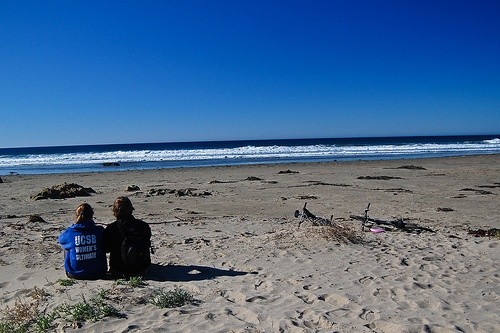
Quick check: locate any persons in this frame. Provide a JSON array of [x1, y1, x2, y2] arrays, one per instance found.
[[107, 196, 152, 280], [59, 203, 111, 280]]
